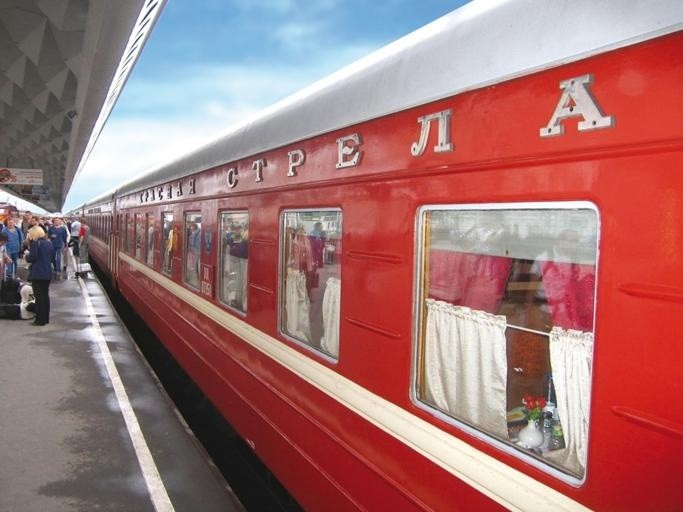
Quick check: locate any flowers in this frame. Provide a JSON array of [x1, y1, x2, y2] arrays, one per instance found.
[[521, 393, 546, 422]]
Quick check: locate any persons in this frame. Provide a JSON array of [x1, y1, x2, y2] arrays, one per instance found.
[[430, 209, 596, 373], [148, 222, 327, 305], [0, 211, 90, 325]]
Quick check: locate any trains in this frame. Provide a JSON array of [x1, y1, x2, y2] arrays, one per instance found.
[[62, 0, 683, 512]]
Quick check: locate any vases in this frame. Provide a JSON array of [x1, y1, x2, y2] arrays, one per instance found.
[[518, 419, 544, 449]]
